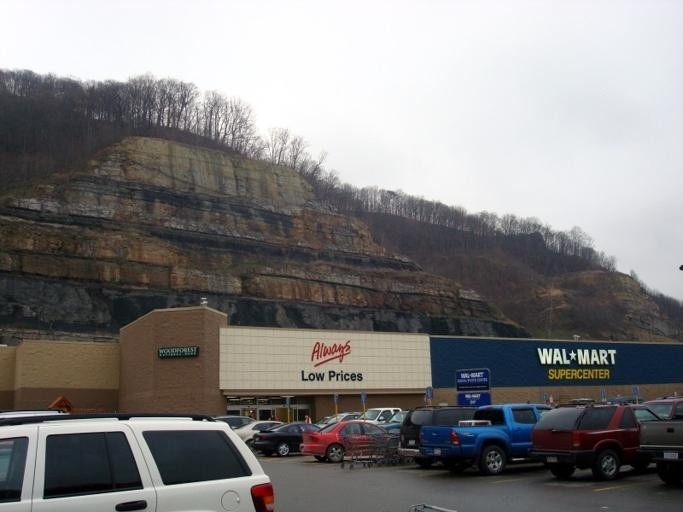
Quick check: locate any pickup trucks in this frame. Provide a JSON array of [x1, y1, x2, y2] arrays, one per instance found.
[[421, 402, 557, 474], [638, 418, 683, 488]]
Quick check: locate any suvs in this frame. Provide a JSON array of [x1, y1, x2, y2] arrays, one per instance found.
[[398, 404, 476, 470], [0, 408, 71, 417], [0, 412, 276, 512], [529, 398, 667, 479]]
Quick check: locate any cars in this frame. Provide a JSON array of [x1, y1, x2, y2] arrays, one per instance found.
[[640, 398, 683, 419], [216, 406, 408, 464]]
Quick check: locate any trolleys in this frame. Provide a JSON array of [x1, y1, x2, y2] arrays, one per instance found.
[[338, 432, 400, 470]]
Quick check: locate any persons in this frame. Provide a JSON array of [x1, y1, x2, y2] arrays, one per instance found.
[[302, 414, 312, 424]]
